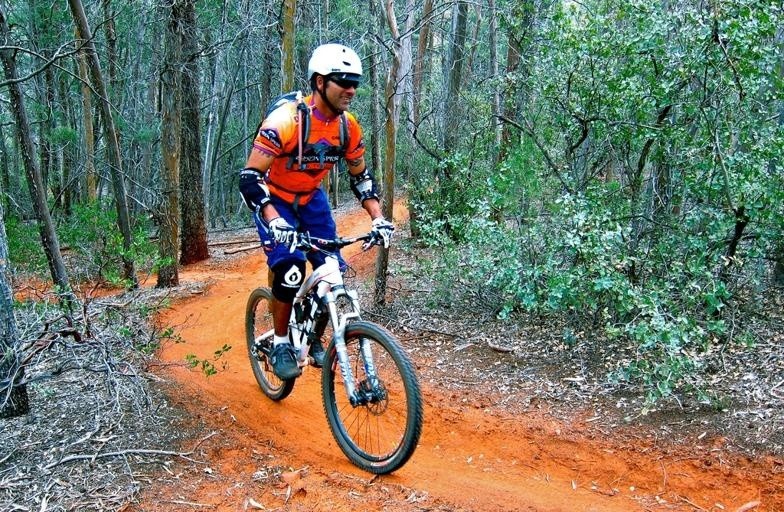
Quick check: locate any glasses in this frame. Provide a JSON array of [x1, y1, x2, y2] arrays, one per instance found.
[[328, 77, 359, 89]]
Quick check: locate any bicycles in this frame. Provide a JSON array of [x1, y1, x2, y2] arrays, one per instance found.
[[240, 227, 425, 476]]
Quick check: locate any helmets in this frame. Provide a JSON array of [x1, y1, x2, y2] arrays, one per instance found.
[[307, 43, 363, 82]]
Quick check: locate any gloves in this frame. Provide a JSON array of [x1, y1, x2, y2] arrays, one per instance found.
[[268, 217, 298, 255], [370, 216, 395, 249]]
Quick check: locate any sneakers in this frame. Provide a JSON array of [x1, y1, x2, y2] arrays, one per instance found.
[[269, 342, 301, 380], [308, 337, 326, 368]]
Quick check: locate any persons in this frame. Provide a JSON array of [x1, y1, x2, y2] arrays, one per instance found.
[[236, 42, 396, 382]]
